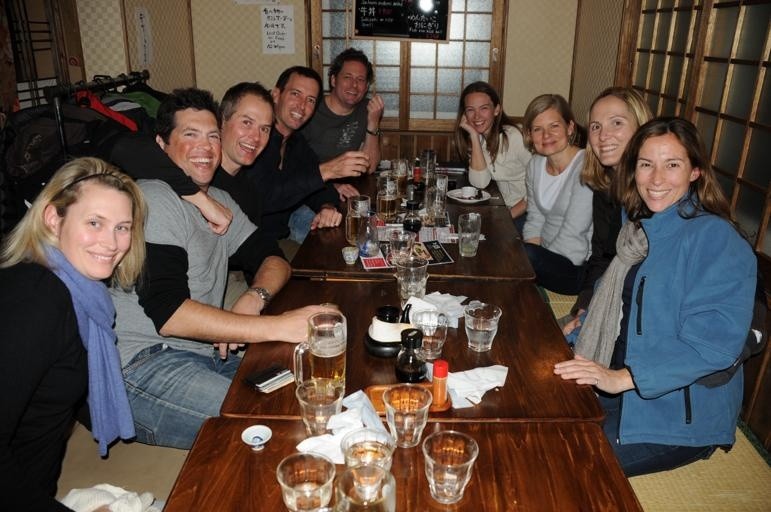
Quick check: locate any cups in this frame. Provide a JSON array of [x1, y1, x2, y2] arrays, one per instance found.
[[422, 429, 479, 504], [345, 194, 370, 246], [421, 152, 438, 173], [276, 451, 336, 512], [383, 384, 433, 449], [426, 188, 447, 219], [296, 378, 345, 437], [389, 231, 416, 264], [458, 214, 481, 257], [294, 311, 347, 397], [342, 247, 359, 265], [241, 425, 272, 453], [413, 309, 449, 361], [336, 464, 396, 512], [377, 193, 398, 221], [392, 158, 408, 180], [377, 176, 398, 196], [433, 173, 448, 195], [465, 301, 502, 352], [340, 428, 396, 482], [397, 256, 429, 297]]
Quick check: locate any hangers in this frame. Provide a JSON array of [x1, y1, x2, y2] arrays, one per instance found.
[[105, 76, 118, 92], [130, 71, 140, 84], [79, 80, 91, 108], [117, 73, 131, 87]]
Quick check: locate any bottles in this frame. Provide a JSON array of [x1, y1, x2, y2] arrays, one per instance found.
[[413, 160, 426, 201], [395, 328, 428, 383], [403, 200, 422, 243], [432, 360, 449, 405]]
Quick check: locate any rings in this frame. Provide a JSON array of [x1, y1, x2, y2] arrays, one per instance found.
[[595, 378, 599, 385]]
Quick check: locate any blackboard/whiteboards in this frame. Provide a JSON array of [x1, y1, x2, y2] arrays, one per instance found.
[[351, 0, 453, 44]]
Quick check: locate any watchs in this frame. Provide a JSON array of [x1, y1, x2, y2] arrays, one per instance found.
[[365, 128, 381, 137], [246, 287, 272, 308]]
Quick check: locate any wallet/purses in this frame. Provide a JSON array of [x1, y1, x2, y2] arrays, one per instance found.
[[242, 366, 295, 394]]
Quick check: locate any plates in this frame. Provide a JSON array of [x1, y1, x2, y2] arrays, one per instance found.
[[446, 189, 491, 203], [379, 169, 414, 179]]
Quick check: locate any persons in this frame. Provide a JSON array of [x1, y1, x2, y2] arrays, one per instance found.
[[452, 81, 534, 219], [253, 66, 370, 246], [556, 86, 655, 346], [300, 47, 384, 202], [146, 81, 280, 313], [104, 87, 292, 450], [552, 115, 758, 479], [0, 156, 147, 512], [519, 93, 594, 296]]
